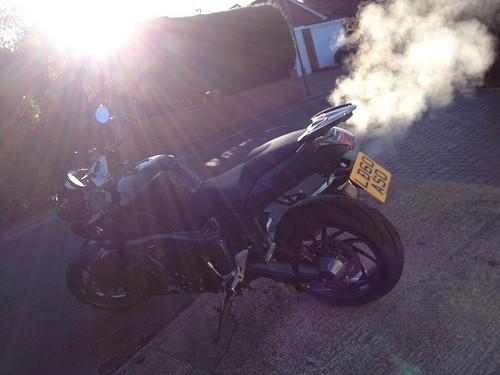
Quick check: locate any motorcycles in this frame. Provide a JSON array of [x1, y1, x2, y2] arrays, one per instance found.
[[58, 102, 404, 341]]
